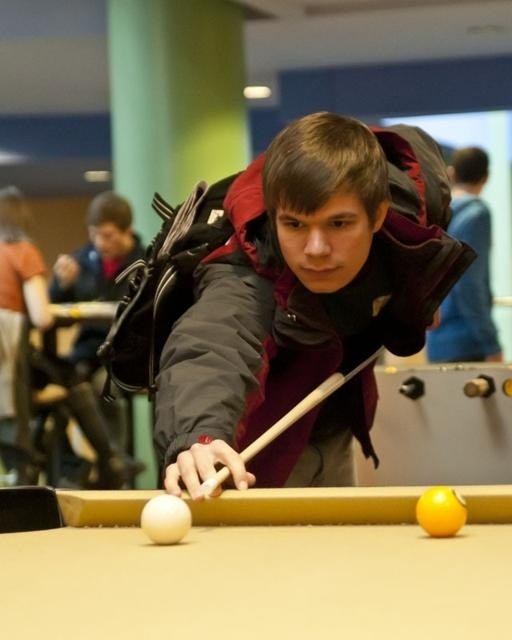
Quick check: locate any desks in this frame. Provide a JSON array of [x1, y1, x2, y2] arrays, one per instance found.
[[0, 484, 512, 640]]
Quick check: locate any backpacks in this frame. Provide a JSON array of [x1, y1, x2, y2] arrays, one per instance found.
[[99, 166, 248, 405]]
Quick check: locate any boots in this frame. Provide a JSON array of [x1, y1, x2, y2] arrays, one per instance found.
[[70, 384, 147, 486]]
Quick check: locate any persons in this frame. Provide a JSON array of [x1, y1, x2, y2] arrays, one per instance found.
[[0, 186, 146, 490], [152, 111, 478, 504], [426, 147, 503, 362], [50, 191, 144, 375]]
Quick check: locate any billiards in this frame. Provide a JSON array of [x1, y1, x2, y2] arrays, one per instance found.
[[417, 489, 466, 538], [142, 495, 191, 544]]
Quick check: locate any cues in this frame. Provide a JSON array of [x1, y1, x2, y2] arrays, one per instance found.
[[201, 341, 384, 497]]
